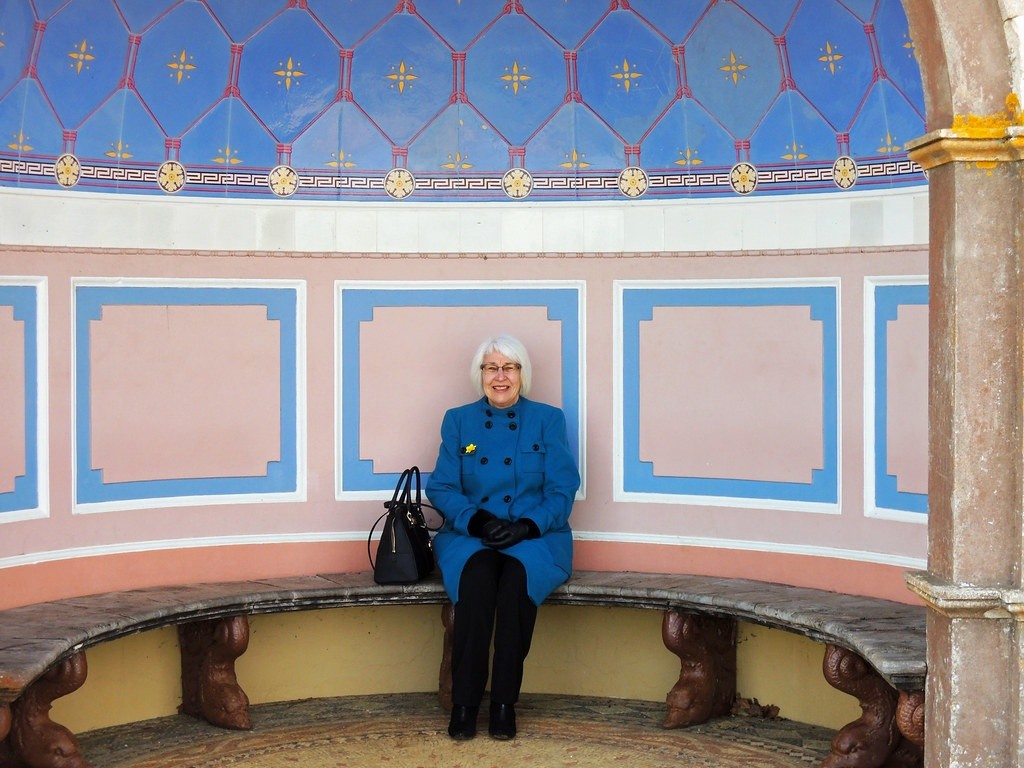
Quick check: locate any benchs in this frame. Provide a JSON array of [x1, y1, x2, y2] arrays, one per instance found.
[[0, 568, 926, 768]]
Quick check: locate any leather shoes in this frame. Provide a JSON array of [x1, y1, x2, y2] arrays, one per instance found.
[[448, 702, 479, 741], [489, 703, 517, 740]]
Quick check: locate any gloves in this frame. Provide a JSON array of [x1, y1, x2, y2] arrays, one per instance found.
[[468, 508, 501, 539], [481, 516, 541, 549]]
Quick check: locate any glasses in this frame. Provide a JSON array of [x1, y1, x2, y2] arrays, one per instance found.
[[480, 363, 520, 376]]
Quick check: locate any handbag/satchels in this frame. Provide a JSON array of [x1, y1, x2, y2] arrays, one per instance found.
[[368, 466, 445, 585]]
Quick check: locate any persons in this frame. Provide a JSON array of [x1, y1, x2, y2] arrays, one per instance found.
[[425, 333, 581, 741]]
